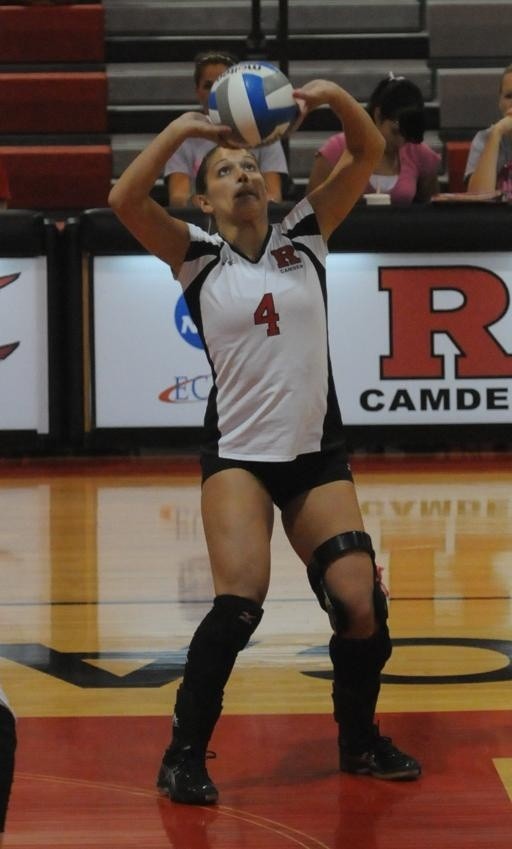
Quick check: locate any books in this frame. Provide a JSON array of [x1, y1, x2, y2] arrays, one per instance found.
[[429, 191, 503, 203]]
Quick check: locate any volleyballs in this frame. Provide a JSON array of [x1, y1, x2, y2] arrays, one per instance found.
[[208, 61, 297, 149]]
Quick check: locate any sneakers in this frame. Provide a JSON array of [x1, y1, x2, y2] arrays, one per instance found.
[[336, 728, 424, 782], [153, 748, 223, 808]]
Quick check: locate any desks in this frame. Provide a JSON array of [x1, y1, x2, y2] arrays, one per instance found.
[[74, 198, 510, 451]]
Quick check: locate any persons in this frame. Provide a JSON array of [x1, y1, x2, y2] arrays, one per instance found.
[[301, 69, 442, 202], [163, 54, 291, 205], [462, 67, 512, 201], [106, 68, 421, 807]]
